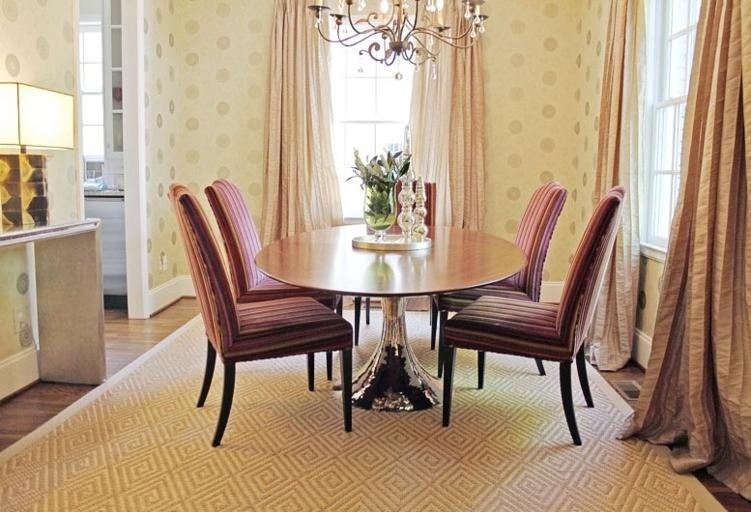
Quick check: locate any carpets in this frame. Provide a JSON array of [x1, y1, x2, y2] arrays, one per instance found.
[[0, 306, 727, 512]]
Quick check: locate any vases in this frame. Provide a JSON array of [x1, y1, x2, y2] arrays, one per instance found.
[[358, 180, 397, 241]]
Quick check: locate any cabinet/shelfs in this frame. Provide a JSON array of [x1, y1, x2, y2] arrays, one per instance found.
[[101, 0, 124, 172]]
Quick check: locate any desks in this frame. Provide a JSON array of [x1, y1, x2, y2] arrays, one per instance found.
[[255, 218, 527, 414], [0, 214, 109, 403]]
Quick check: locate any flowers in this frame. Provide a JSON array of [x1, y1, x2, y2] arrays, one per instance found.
[[346, 147, 412, 241]]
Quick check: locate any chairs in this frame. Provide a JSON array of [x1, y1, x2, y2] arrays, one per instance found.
[[355, 180, 437, 350]]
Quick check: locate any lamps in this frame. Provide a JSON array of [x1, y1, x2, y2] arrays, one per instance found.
[[0, 81, 74, 230], [309, 0, 489, 81]]
